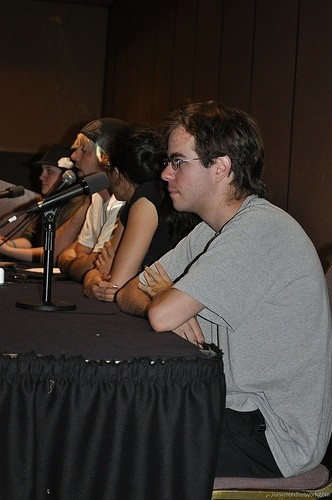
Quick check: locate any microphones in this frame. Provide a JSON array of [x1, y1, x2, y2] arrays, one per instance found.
[[0, 185, 25, 198], [30, 170, 76, 221], [27, 172, 111, 214]]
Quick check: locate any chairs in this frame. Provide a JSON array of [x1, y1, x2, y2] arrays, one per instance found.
[[212, 241, 332, 500]]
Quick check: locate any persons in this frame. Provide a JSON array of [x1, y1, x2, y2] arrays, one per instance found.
[[115, 101, 331, 478], [0, 117, 201, 303]]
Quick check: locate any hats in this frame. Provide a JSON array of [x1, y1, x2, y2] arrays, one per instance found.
[[79, 117, 130, 157], [32, 145, 76, 171]]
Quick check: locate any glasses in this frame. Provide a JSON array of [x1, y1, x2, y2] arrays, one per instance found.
[[163, 153, 226, 170]]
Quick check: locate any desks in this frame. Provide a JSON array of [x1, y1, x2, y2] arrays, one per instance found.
[[0, 252, 227, 500]]
